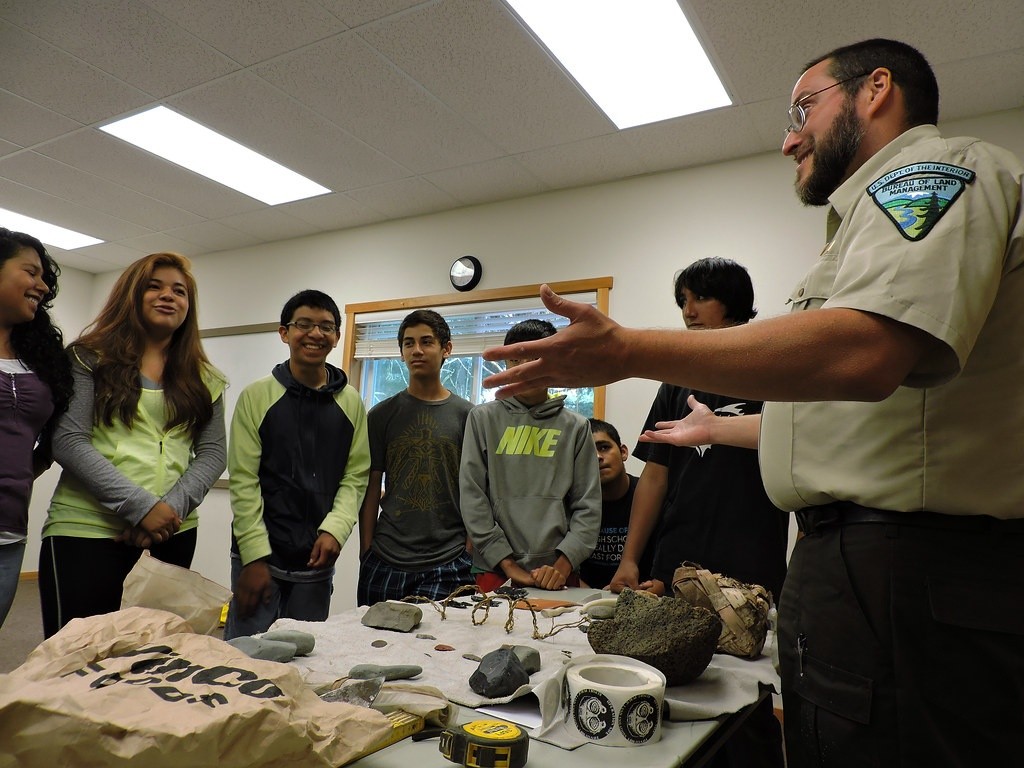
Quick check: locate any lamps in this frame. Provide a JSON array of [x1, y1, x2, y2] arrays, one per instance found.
[[448, 255, 482, 292]]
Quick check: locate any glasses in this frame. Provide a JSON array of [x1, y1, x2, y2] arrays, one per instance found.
[[784, 72, 872, 134], [286, 322, 338, 335]]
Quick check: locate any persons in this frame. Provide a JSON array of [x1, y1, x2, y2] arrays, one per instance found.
[[484, 37, 1024, 768], [0, 225, 791, 639]]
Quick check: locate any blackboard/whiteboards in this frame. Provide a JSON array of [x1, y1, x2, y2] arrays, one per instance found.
[[195, 321, 292, 491]]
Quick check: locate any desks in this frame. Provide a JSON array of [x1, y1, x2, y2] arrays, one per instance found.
[[252, 584, 786, 768]]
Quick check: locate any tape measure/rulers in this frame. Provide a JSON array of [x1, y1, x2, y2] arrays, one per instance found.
[[412, 721, 530, 768]]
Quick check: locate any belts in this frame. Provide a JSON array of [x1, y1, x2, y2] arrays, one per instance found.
[[792, 501, 1024, 535]]
